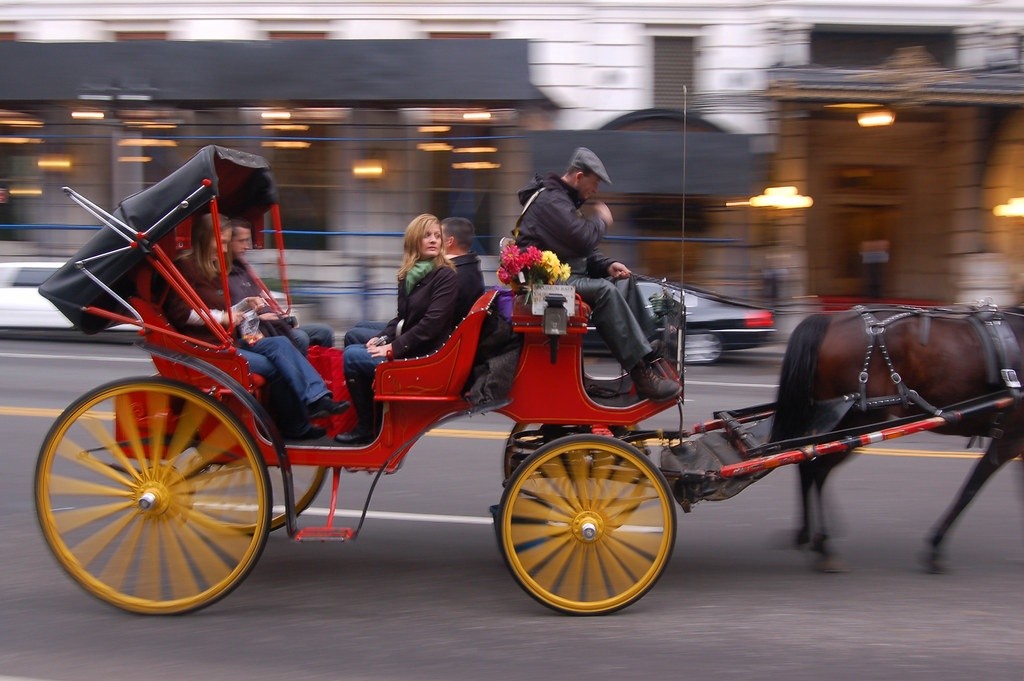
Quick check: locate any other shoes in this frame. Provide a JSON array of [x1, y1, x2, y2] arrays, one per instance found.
[[335, 431, 370, 444]]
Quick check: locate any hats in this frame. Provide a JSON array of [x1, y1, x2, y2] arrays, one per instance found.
[[572, 147, 612, 184]]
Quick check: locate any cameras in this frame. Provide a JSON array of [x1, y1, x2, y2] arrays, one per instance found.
[[373, 335, 388, 346]]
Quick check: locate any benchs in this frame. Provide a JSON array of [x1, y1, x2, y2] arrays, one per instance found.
[[375, 289, 500, 396], [129, 298, 266, 391], [499, 237, 594, 336]]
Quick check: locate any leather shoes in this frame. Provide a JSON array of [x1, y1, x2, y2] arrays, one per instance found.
[[634, 368, 683, 402], [650, 338, 676, 363]]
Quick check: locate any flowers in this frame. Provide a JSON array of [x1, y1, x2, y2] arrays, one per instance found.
[[498, 245, 571, 305]]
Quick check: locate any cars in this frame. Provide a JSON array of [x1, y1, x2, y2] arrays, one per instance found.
[[0, 259, 296, 342], [565, 269, 776, 365]]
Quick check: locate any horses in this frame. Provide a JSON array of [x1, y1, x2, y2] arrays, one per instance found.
[[770, 299, 1024, 575]]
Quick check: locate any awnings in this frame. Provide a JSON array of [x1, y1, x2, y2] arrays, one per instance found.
[[0, 38, 562, 107], [525, 130, 780, 193]]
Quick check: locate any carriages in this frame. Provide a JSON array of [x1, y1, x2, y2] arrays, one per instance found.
[[32, 141, 1024, 616]]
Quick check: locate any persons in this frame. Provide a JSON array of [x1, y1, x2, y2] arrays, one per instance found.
[[511, 145, 683, 403], [163, 212, 351, 440], [334, 212, 485, 443]]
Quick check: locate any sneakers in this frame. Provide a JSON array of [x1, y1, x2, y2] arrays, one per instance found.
[[307, 395, 350, 418], [296, 426, 327, 441]]
